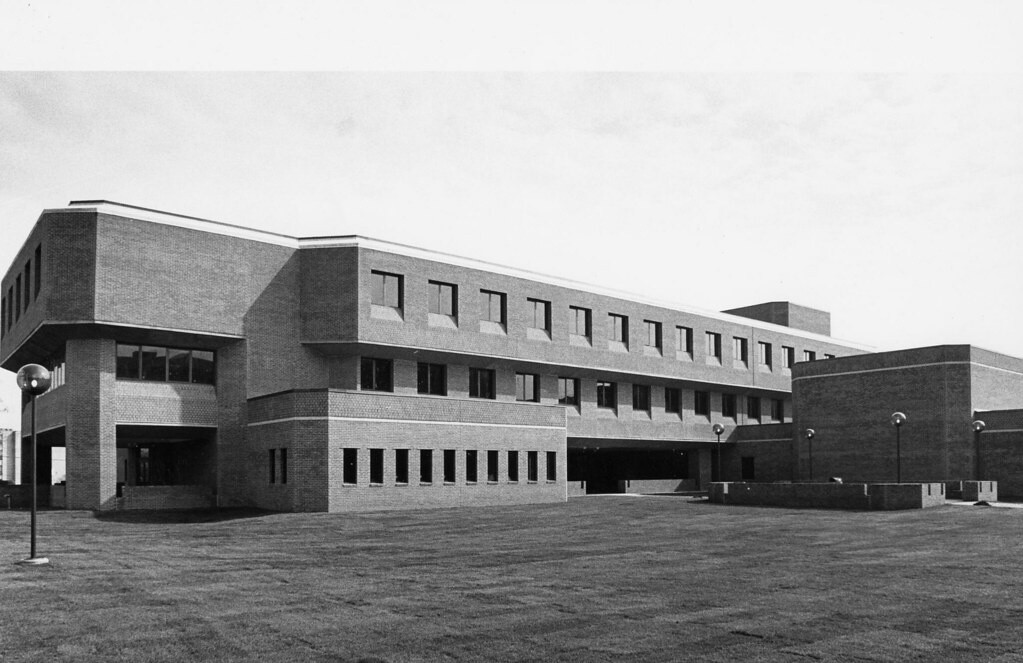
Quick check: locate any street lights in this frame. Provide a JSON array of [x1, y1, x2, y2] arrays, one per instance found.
[[971, 420, 986, 482], [17, 364, 52, 567], [891, 412, 907, 485], [712, 423, 725, 481], [806, 428, 815, 483]]
